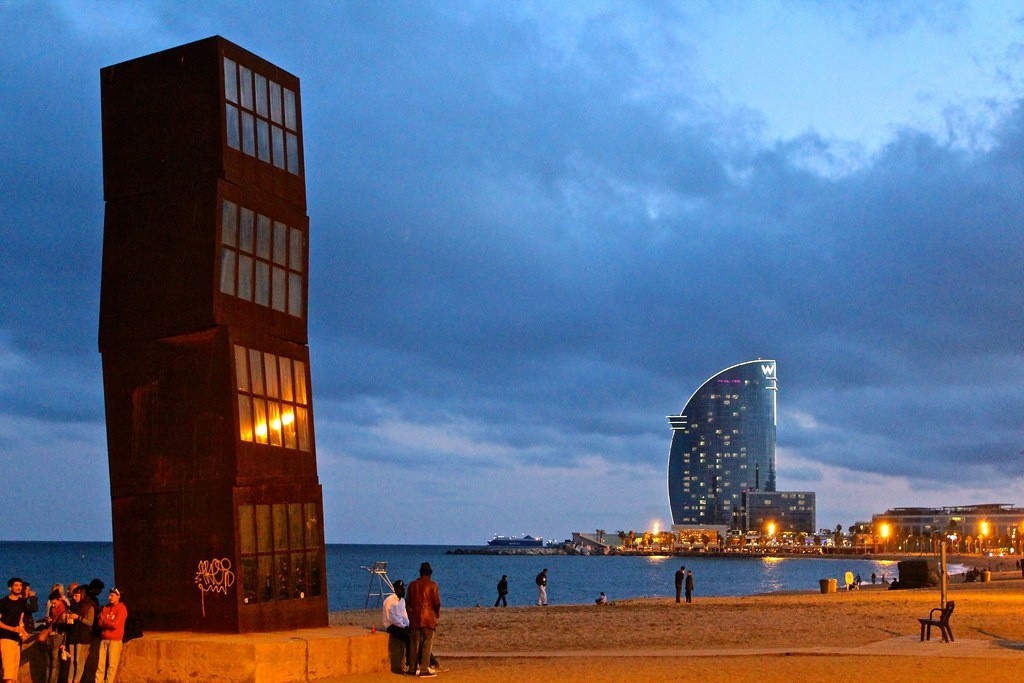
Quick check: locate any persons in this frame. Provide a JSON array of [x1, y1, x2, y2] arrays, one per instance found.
[[685, 570, 694, 603], [382, 563, 441, 678], [964, 558, 1024, 583], [675, 566, 685, 603], [494, 575, 509, 607], [853, 572, 898, 591], [0, 578, 128, 683], [536, 569, 548, 605], [596, 592, 608, 604]]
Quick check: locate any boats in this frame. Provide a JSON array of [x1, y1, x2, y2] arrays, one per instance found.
[[546, 540, 566, 548], [487, 534, 543, 547]]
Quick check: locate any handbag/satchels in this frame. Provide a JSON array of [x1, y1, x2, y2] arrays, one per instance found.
[[596, 599, 599, 602], [36, 629, 52, 642], [61, 648, 67, 661]]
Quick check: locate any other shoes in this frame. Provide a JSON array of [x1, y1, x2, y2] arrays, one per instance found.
[[389, 666, 451, 677]]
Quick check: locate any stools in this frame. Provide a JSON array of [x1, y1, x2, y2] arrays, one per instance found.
[[365, 560, 394, 610]]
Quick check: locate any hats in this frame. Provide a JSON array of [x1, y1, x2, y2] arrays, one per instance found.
[[392, 580, 408, 588], [419, 562, 433, 574], [110, 588, 120, 595]]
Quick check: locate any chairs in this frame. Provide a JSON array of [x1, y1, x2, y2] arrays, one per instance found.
[[918, 601, 956, 643]]
